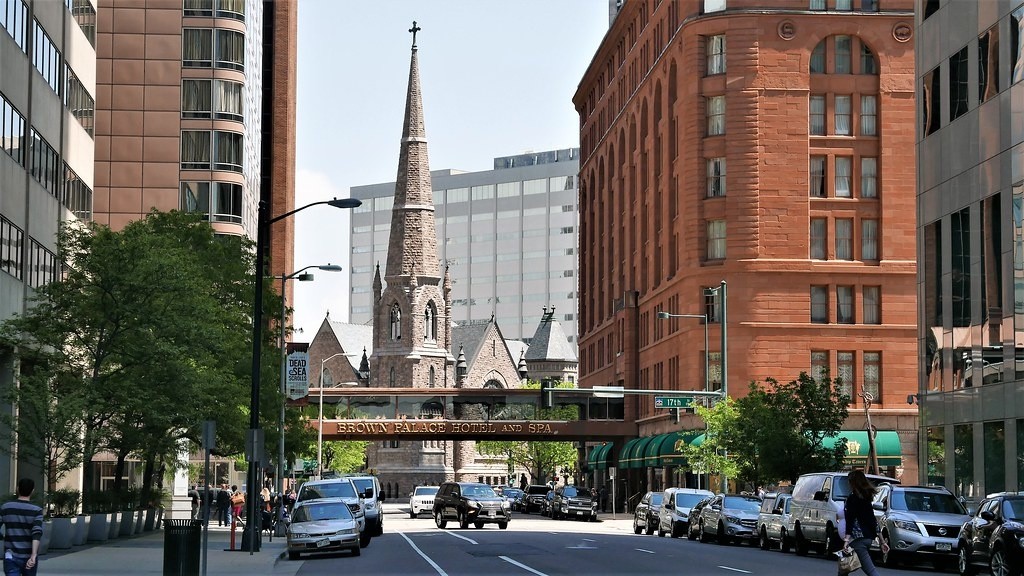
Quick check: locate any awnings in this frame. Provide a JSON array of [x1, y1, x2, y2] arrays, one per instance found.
[[588, 430, 903, 470]]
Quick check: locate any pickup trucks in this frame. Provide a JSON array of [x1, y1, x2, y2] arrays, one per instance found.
[[757, 492, 793, 553]]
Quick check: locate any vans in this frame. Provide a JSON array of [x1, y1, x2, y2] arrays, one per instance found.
[[343, 477, 386, 538]]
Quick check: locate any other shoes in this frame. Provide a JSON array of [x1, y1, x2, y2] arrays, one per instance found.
[[225, 525, 227, 527], [242, 524, 246, 530], [230, 528, 237, 530], [219, 524, 221, 526]]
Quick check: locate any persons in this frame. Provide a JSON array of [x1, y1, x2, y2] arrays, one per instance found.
[[838, 471, 890, 576], [758, 487, 766, 499], [189, 484, 297, 527], [0, 478, 43, 576], [520, 472, 528, 490], [591, 488, 596, 496]]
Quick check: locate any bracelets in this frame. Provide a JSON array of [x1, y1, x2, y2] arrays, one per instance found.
[[881, 542, 886, 544], [844, 538, 849, 542]]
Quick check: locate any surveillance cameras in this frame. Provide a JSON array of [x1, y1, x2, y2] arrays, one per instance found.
[[907, 395, 914, 405]]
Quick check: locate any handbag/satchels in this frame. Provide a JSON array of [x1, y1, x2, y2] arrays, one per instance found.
[[837, 546, 862, 575], [231, 492, 245, 506]]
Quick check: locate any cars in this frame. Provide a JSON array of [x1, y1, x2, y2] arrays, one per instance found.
[[282, 496, 362, 561], [698, 492, 762, 547], [687, 495, 714, 540], [633, 491, 665, 536], [491, 483, 525, 512], [542, 490, 554, 517]]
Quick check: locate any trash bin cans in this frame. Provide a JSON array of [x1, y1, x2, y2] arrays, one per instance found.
[[159, 518, 203, 576]]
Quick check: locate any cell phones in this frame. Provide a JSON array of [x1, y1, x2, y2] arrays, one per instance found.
[[5, 552, 12, 559], [844, 547, 853, 555]]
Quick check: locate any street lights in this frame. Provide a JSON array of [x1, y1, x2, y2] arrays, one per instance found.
[[214, 456, 220, 488], [238, 196, 363, 552], [273, 259, 343, 539], [315, 351, 357, 492], [658, 312, 711, 494], [710, 279, 730, 494]]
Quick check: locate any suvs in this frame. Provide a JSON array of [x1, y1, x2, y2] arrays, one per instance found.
[[952, 490, 1024, 576], [657, 487, 717, 538], [787, 471, 902, 560], [409, 485, 440, 519], [431, 481, 512, 530], [866, 482, 974, 569], [550, 486, 598, 523], [520, 484, 554, 515], [282, 478, 370, 549]]
